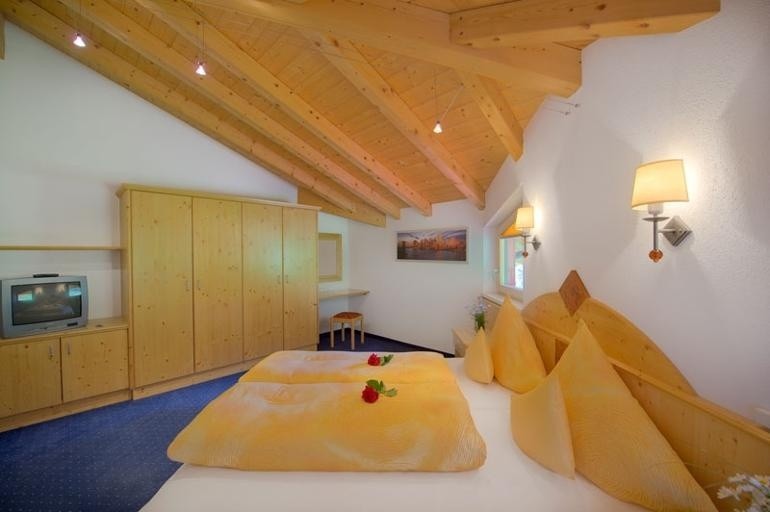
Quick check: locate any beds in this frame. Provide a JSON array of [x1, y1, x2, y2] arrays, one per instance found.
[[137, 269, 768, 512]]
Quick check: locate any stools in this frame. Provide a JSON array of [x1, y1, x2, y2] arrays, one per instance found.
[[329, 311, 365, 350]]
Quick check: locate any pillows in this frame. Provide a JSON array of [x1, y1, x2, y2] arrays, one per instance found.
[[463, 326, 495, 384], [486, 295, 547, 395], [549, 317, 719, 512], [510, 370, 577, 481]]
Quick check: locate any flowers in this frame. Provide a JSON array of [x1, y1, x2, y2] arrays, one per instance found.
[[463, 292, 488, 331]]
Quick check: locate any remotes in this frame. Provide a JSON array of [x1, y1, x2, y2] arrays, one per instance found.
[[33, 273, 58, 278]]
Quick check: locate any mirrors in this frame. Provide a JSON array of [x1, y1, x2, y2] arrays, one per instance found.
[[317, 231, 342, 281]]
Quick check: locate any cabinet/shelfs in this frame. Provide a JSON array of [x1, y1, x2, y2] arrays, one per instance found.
[[115, 183, 324, 402], [1, 315, 131, 434]]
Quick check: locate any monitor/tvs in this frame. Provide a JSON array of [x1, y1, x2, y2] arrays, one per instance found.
[[0, 275, 89, 339]]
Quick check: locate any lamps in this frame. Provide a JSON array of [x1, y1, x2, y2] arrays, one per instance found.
[[630, 155, 693, 263], [515, 206, 542, 257]]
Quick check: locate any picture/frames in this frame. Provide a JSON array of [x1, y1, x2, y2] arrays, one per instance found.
[[394, 225, 469, 264]]
